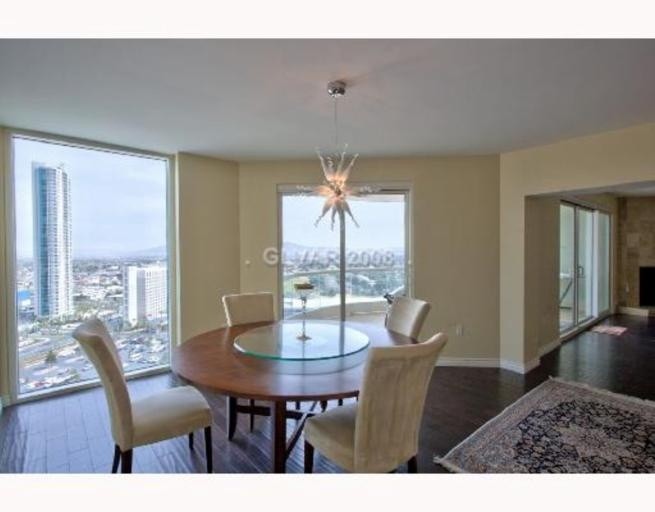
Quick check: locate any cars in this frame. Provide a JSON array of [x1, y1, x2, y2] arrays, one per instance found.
[[18, 326, 168, 393]]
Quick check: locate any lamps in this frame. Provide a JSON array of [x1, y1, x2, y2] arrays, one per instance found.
[[296, 78, 381, 233]]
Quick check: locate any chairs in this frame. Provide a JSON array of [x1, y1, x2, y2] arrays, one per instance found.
[[71, 316, 215, 473], [221, 292, 275, 326], [303, 294, 448, 474]]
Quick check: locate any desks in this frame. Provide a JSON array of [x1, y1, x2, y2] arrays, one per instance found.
[[169, 319, 420, 474]]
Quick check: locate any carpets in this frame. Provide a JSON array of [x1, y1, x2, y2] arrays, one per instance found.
[[431, 373, 655, 473], [587, 324, 627, 337]]
